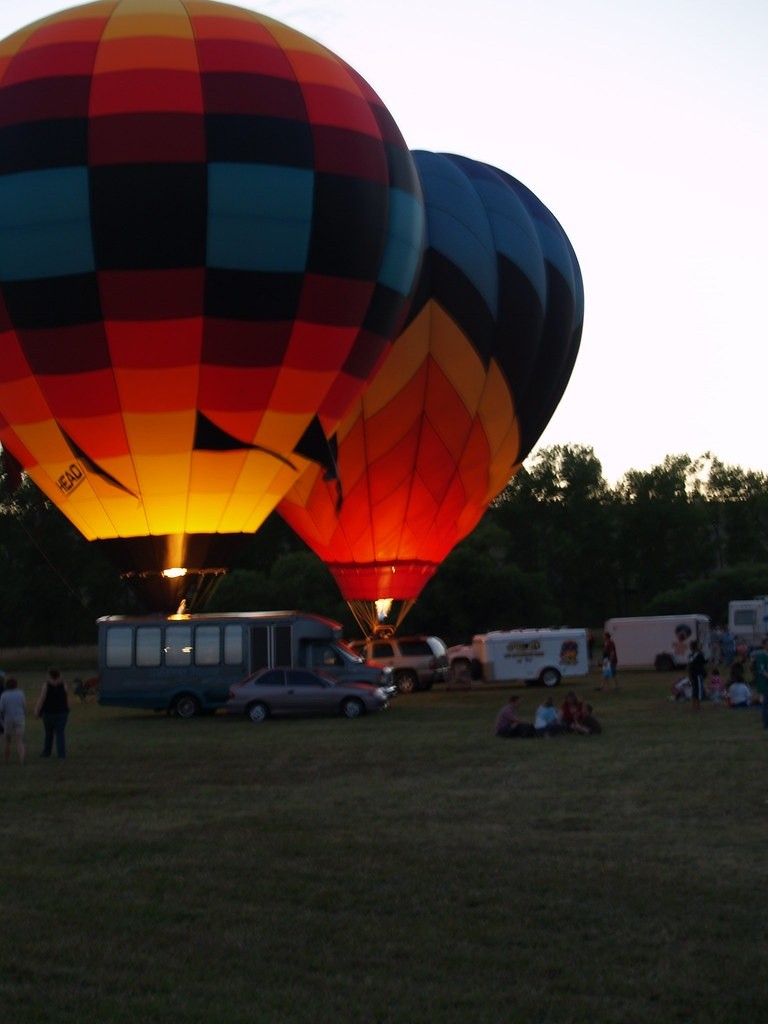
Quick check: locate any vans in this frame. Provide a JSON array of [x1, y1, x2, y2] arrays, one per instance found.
[[347, 636, 449, 694]]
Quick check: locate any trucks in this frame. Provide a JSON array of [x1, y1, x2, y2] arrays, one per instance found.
[[447, 629, 588, 688], [604, 615, 713, 673], [95, 611, 398, 718]]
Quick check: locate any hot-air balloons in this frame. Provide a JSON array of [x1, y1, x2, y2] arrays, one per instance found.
[[0, 0, 423, 617], [274, 150, 585, 641]]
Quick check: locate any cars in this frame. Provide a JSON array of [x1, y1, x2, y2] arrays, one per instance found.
[[228, 667, 388, 723]]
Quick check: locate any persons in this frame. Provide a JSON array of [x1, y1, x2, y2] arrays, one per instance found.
[[535, 696, 559, 738], [34, 668, 69, 760], [496, 694, 543, 737], [600, 632, 618, 691], [0, 674, 28, 766], [674, 625, 768, 715], [557, 691, 601, 737]]
